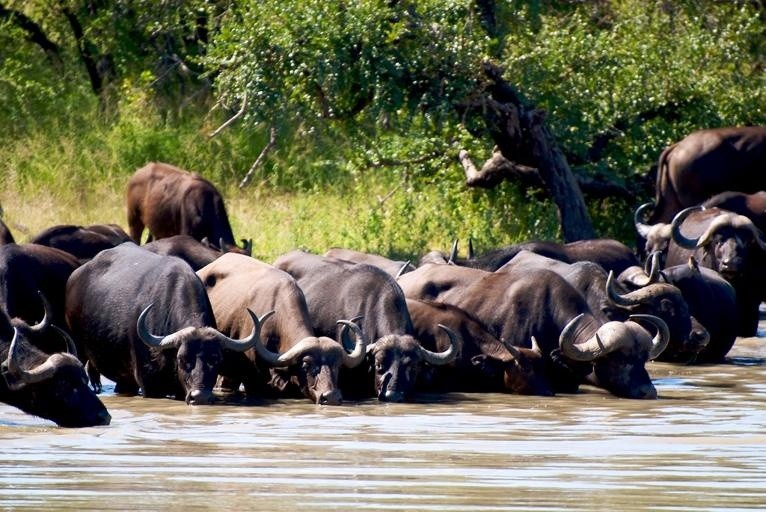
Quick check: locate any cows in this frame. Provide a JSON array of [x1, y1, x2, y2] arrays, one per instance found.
[[649, 126, 765, 224], [123, 160, 253, 257], [0, 191, 765, 427]]
